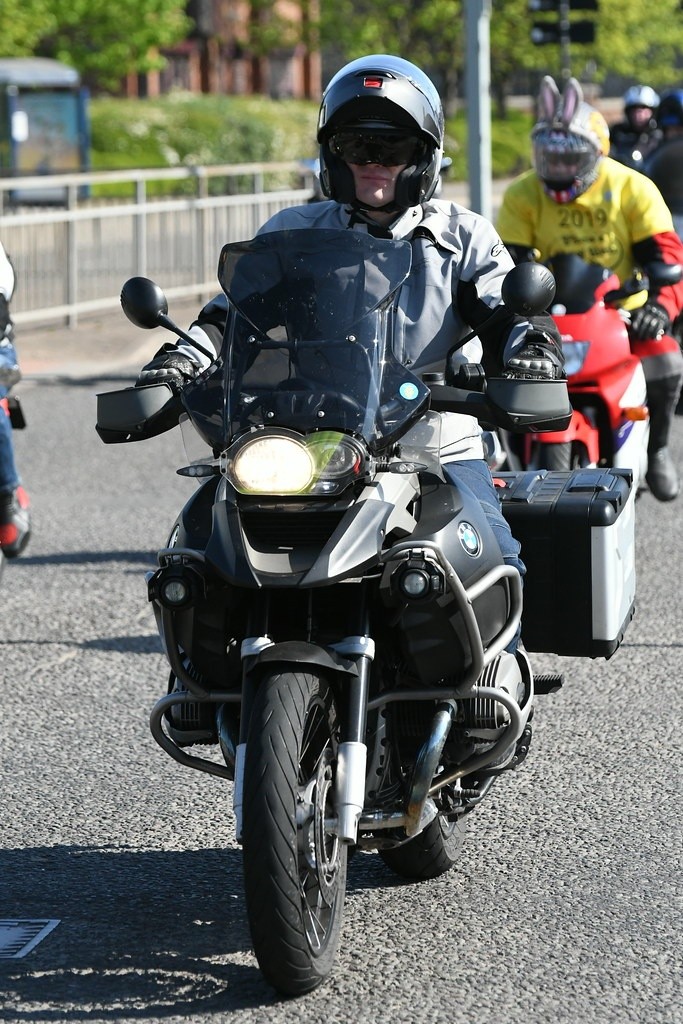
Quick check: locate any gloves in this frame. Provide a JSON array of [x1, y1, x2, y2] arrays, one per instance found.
[[133, 352, 196, 392], [504, 346, 559, 380], [630, 304, 669, 342]]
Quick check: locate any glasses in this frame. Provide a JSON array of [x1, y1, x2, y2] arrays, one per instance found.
[[324, 120, 427, 167]]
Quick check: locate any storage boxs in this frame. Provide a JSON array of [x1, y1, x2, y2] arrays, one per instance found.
[[487, 467, 639, 662]]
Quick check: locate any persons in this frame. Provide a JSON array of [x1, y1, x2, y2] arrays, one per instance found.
[[2, 239, 33, 555], [610, 85, 682, 236], [135, 53, 526, 742], [496, 75, 683, 502]]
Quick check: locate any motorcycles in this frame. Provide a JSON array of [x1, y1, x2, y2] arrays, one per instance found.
[[496, 234, 683, 516], [109, 258, 559, 992]]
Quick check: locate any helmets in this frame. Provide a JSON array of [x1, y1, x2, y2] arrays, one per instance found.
[[530, 96, 610, 203], [316, 54, 445, 207], [622, 85, 660, 110]]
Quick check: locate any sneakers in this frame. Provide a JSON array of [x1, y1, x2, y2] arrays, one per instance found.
[[0, 485, 32, 558]]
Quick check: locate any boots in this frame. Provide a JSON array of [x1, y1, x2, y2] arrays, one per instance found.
[[645, 374, 682, 501]]
